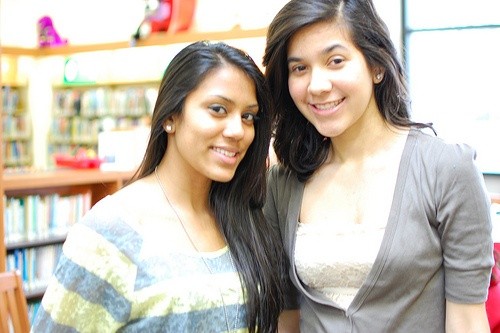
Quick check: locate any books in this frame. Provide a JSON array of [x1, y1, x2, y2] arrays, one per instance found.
[[6, 189, 92, 333], [3, 86, 149, 163]]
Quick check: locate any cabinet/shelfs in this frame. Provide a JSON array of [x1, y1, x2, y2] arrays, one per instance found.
[[1, 25, 270, 332]]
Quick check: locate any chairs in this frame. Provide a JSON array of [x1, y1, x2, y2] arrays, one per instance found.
[[0, 269, 31, 332]]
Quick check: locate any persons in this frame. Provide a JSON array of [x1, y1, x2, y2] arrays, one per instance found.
[[31, 44, 279, 333], [263, 0, 493, 332]]
